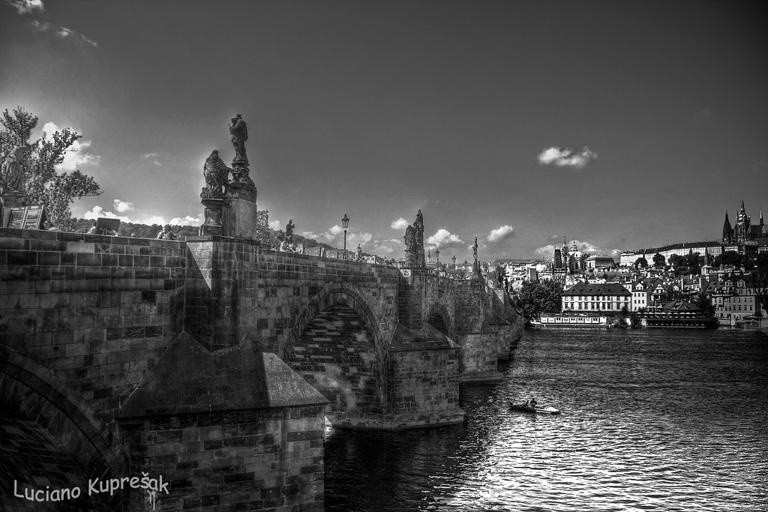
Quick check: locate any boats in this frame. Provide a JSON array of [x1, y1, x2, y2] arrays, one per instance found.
[[510, 396, 561, 416]]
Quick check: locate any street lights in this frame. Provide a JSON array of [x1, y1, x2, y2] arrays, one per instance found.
[[452, 252, 457, 271], [433, 247, 441, 274], [340, 210, 352, 260]]
